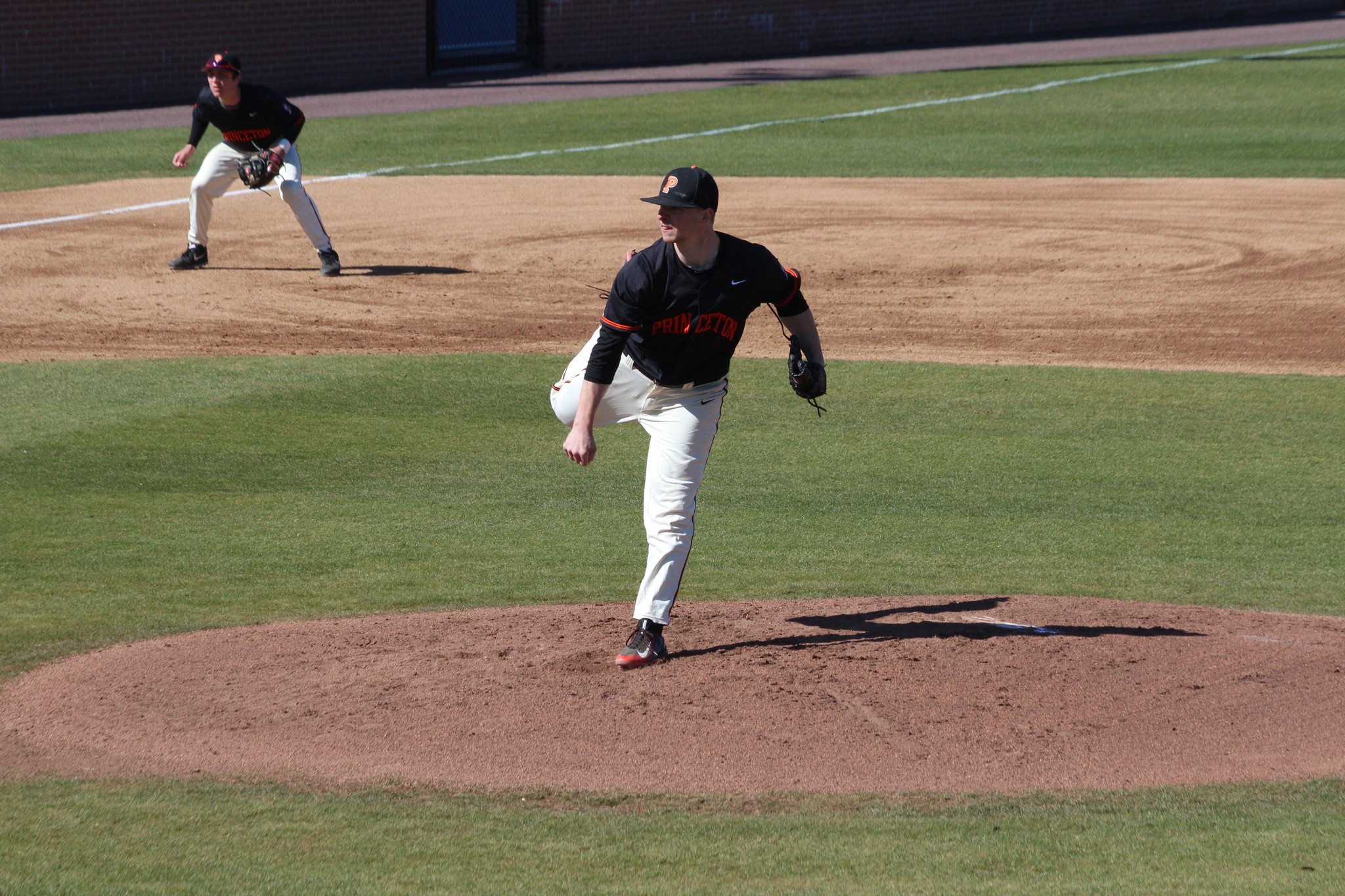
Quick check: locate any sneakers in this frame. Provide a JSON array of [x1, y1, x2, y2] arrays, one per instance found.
[[318, 250, 341, 277], [169, 245, 209, 270], [615, 618, 667, 669], [585, 250, 637, 299]]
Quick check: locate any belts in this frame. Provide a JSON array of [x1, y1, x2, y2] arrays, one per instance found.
[[623, 350, 727, 390]]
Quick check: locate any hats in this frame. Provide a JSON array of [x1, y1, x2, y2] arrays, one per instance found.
[[201, 51, 242, 74], [640, 165, 719, 211]]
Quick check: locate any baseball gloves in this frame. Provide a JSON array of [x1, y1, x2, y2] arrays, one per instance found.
[[786, 337, 827, 399], [234, 146, 288, 190]]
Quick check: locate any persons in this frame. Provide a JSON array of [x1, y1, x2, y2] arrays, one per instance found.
[[551, 167, 828, 666], [166, 51, 342, 276]]
